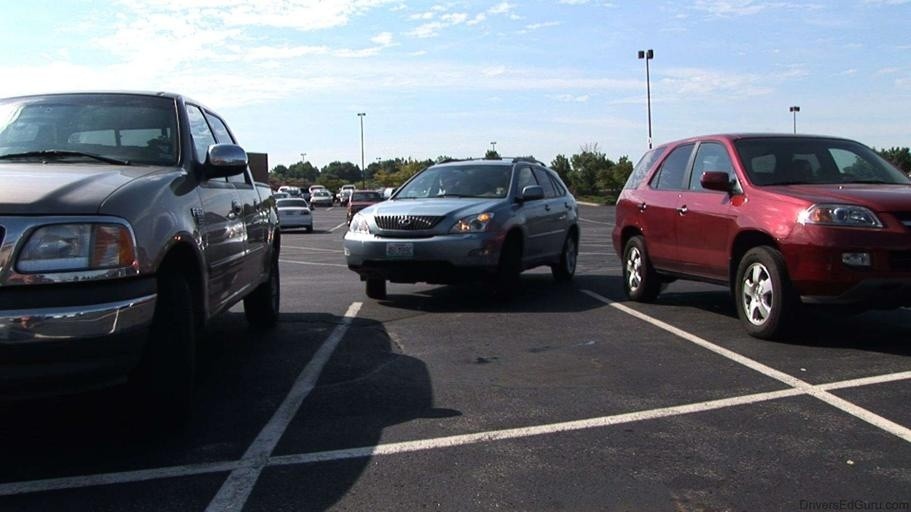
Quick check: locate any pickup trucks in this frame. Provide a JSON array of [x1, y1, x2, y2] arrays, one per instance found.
[[0, 89, 283, 407]]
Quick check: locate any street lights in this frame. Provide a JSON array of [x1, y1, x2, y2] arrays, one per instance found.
[[788, 105, 801, 133], [358, 112, 368, 189], [638, 48, 655, 151], [300, 152, 306, 163], [490, 142, 496, 150]]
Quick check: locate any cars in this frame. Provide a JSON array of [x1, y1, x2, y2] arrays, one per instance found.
[[272, 184, 397, 233], [343, 159, 580, 298]]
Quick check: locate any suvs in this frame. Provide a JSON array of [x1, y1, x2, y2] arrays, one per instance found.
[[609, 131, 910, 341]]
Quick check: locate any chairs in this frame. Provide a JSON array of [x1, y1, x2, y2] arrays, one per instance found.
[[790, 158, 813, 183]]
